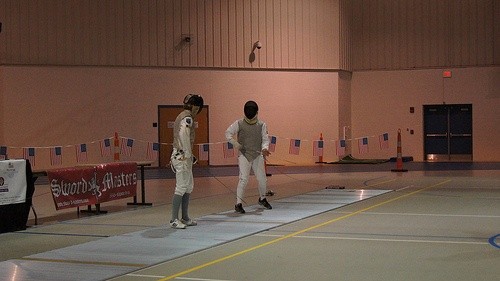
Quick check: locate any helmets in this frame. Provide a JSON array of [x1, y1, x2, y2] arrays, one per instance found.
[[244, 101, 259, 120], [187, 95, 204, 115]]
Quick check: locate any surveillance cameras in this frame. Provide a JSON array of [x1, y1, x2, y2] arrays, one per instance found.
[[256, 43, 262, 49]]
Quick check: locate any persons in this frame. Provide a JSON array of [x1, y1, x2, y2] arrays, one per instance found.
[[169, 94, 204, 230], [224, 101, 273, 215]]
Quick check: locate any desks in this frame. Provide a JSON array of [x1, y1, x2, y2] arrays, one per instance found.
[[0, 160, 33, 234], [28, 161, 155, 226]]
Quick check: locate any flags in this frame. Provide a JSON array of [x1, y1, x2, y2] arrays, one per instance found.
[[99, 138, 111, 159], [358, 138, 369, 154], [0, 146, 10, 161], [49, 145, 62, 166], [22, 148, 37, 167], [145, 143, 160, 161], [312, 141, 323, 157], [222, 142, 234, 158], [267, 137, 276, 153], [120, 138, 133, 156], [378, 133, 389, 149], [335, 139, 345, 157], [75, 143, 89, 162], [198, 144, 209, 162], [288, 138, 301, 156]]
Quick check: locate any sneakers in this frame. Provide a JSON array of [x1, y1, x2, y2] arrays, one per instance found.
[[181, 217, 198, 226], [169, 219, 186, 229], [258, 197, 273, 209], [235, 203, 245, 214]]
